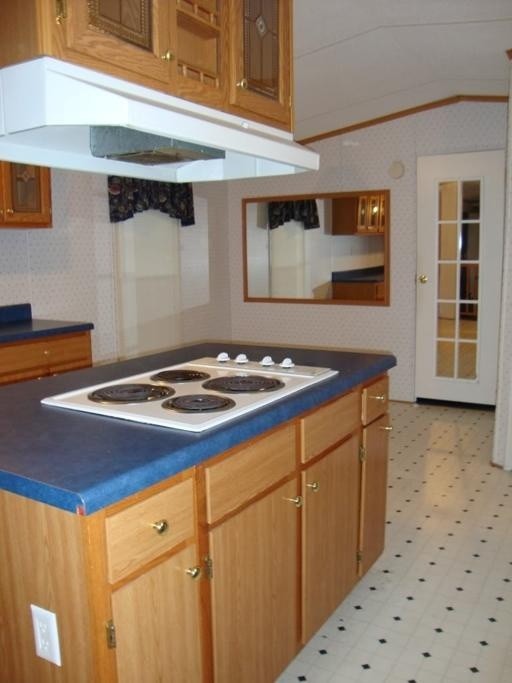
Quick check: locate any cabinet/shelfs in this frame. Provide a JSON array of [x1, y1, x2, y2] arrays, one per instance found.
[[0, 1, 177, 97], [232, 1, 293, 130], [332, 282, 386, 300], [0, 330, 92, 382], [178, 0, 233, 108], [331, 194, 386, 237], [202, 388, 362, 682], [0, 162, 54, 230], [1, 481, 213, 683], [360, 375, 392, 579]]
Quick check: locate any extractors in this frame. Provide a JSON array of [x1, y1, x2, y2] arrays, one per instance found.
[[0, 55, 321, 185]]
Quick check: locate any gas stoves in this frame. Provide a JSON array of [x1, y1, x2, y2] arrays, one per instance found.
[[40, 355, 340, 433]]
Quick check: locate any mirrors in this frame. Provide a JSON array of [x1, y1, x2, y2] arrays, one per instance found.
[[238, 186, 393, 311]]
